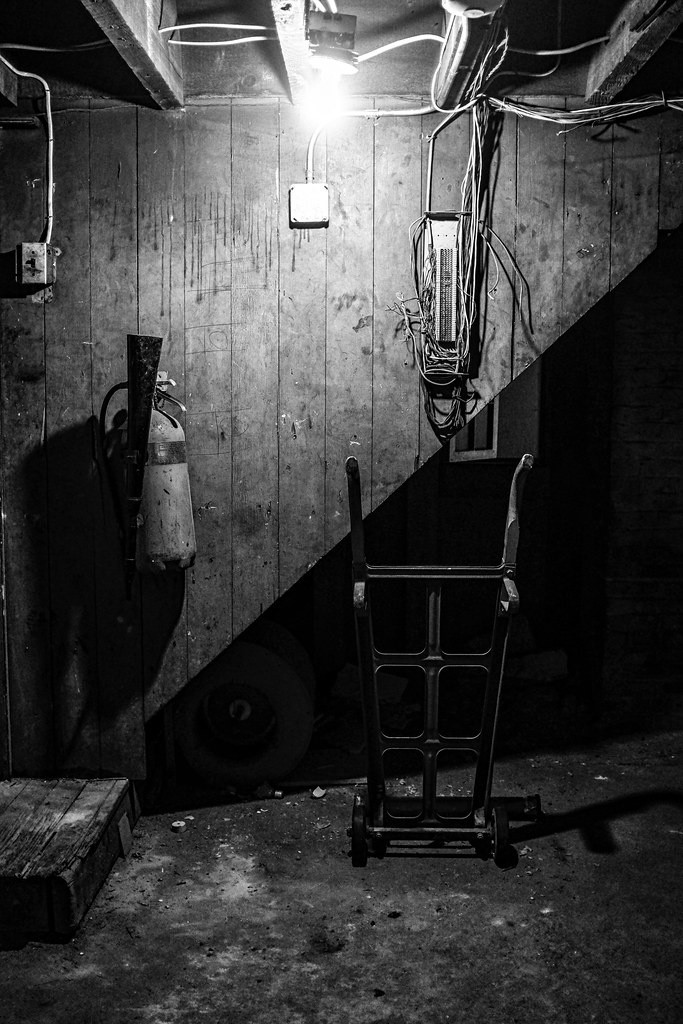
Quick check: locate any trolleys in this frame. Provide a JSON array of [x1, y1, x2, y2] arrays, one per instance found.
[[343, 451, 519, 866]]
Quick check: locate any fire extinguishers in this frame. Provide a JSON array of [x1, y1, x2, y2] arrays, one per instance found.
[[96, 366, 207, 580]]
